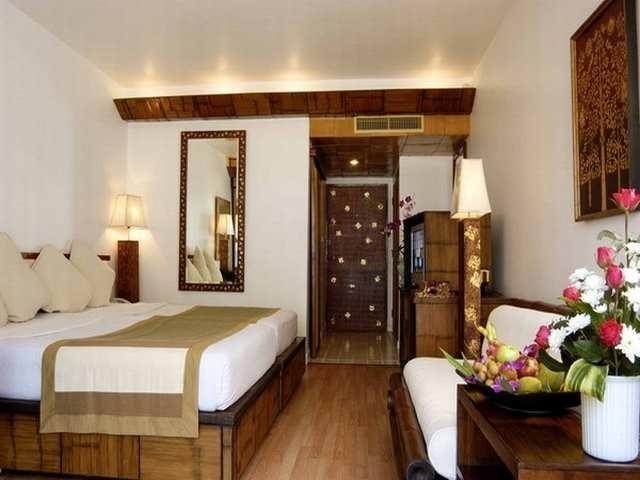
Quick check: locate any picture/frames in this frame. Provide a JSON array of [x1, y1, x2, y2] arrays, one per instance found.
[[215, 196, 230, 260], [570, 0, 640, 221]]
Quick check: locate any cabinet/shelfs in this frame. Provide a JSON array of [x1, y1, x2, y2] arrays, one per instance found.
[[403, 211, 460, 297], [400, 292, 514, 367]]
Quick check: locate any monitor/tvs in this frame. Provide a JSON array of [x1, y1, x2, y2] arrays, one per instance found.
[[411, 223, 425, 281]]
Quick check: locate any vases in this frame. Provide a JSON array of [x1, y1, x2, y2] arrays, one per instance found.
[[581, 375, 640, 463]]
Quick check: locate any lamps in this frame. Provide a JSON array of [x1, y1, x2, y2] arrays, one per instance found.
[[449, 157, 491, 362], [216, 213, 235, 280], [107, 192, 146, 303]]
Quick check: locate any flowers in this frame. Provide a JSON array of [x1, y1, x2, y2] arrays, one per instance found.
[[464, 188, 640, 401]]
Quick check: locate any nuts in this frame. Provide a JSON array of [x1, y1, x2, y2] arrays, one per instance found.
[[474, 355, 503, 386]]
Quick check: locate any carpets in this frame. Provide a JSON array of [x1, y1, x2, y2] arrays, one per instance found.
[[309, 333, 400, 366]]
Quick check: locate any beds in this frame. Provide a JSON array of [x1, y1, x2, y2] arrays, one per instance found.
[[0, 253, 305, 480]]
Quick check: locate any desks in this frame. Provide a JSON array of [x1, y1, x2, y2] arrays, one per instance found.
[[455, 384, 640, 480]]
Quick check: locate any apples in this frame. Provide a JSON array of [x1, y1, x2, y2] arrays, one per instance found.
[[496, 344, 520, 364], [536, 363, 566, 391], [518, 355, 538, 378], [517, 377, 542, 391]]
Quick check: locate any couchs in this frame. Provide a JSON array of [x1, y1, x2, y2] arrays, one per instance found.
[[389, 298, 575, 480]]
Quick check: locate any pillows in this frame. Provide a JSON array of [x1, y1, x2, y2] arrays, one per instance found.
[[186, 258, 205, 284], [204, 249, 223, 286], [29, 245, 92, 313], [70, 239, 116, 308], [0, 295, 8, 329], [192, 245, 212, 284], [0, 232, 48, 323]]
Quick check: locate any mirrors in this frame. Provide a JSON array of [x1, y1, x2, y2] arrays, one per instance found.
[[180, 130, 246, 292]]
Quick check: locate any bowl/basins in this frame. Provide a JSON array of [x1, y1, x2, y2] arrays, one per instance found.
[[454, 364, 579, 418]]
